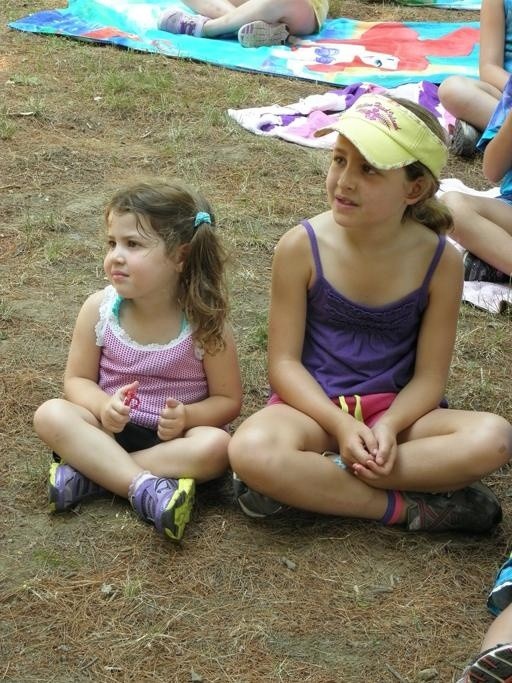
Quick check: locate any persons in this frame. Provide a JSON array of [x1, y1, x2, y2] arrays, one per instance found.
[[455, 550, 512, 682], [438, 75, 511, 283], [437, 0, 511, 155], [31, 175, 244, 540], [227, 90, 511, 534], [156, 0, 331, 46]]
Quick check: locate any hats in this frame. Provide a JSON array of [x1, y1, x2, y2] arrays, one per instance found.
[[314, 93, 449, 179]]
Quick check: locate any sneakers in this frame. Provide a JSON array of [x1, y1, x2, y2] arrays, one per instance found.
[[400, 480, 503, 534], [158, 9, 211, 39], [238, 22, 290, 47], [135, 477, 196, 541], [452, 118, 480, 156], [234, 471, 293, 519], [48, 456, 104, 512], [463, 249, 512, 284]]
